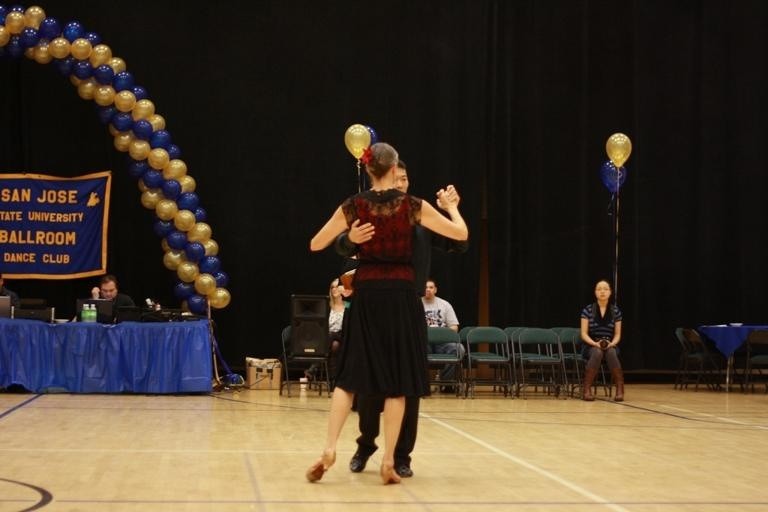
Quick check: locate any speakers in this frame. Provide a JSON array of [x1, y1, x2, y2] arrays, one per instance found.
[[290, 294, 330, 358]]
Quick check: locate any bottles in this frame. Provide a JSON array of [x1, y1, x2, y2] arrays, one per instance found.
[[145, 297, 163, 312], [79, 302, 97, 324]]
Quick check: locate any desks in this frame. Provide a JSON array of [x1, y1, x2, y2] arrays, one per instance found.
[[0, 317, 212, 394], [698, 326, 768, 392]]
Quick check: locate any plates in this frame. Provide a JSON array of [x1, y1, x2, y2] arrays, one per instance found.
[[54, 318, 69, 324]]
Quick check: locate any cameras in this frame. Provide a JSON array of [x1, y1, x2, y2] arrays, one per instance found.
[[595, 337, 611, 348]]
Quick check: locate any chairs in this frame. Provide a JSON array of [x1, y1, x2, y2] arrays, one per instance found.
[[427, 326, 613, 399], [742, 331, 768, 393], [674, 328, 720, 391], [281, 325, 331, 397]]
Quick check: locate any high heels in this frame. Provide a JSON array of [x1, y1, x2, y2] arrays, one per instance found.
[[306, 451, 336, 482], [381, 464, 401, 485]]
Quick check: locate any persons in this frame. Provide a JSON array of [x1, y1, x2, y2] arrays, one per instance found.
[[421, 279, 465, 393], [307, 141, 468, 486], [334, 160, 460, 479], [308, 277, 354, 392], [580, 280, 625, 401]]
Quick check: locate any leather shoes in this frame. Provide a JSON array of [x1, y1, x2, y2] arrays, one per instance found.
[[396, 464, 413, 478], [350, 449, 367, 473]]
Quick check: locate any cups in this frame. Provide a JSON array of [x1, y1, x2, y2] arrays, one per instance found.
[[298, 377, 308, 391]]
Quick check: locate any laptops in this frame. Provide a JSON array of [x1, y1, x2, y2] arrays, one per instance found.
[[76, 299, 113, 324], [0, 295, 11, 318]]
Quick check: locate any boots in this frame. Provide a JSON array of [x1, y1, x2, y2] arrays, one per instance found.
[[610, 367, 623, 401], [582, 368, 596, 400]]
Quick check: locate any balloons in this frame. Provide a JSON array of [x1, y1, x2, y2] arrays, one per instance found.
[[597, 160, 627, 192], [365, 125, 378, 146], [605, 132, 632, 167], [0, 4, 232, 316], [344, 124, 372, 160]]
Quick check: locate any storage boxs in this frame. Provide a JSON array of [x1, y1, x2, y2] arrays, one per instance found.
[[244, 357, 283, 390]]
